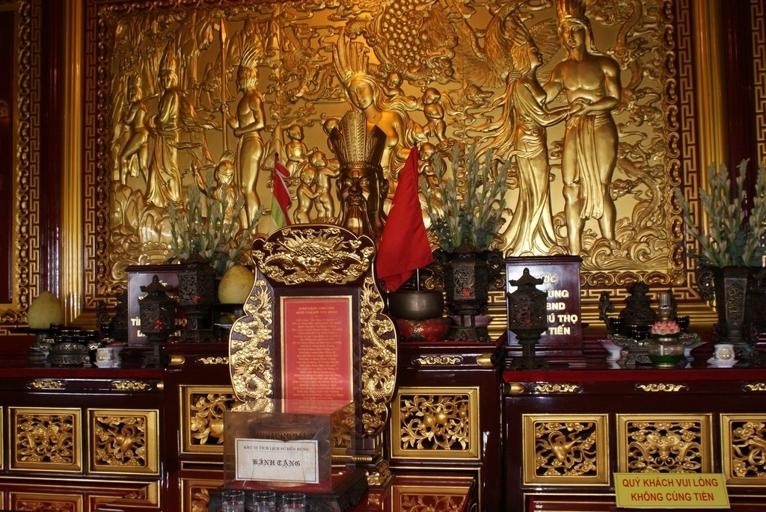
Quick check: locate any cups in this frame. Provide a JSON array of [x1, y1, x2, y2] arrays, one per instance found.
[[714, 343, 736, 361]]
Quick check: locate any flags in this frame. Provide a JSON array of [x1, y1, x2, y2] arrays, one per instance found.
[[375, 147, 433, 294]]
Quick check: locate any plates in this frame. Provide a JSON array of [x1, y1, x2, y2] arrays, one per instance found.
[[711, 359, 739, 369]]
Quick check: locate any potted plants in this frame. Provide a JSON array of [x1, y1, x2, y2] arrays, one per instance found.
[[417, 135, 515, 311], [667, 157, 766, 340]]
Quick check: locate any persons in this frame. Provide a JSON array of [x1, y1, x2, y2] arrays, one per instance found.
[[542, 12, 623, 260], [119, 42, 448, 254], [488, 35, 592, 257]]
